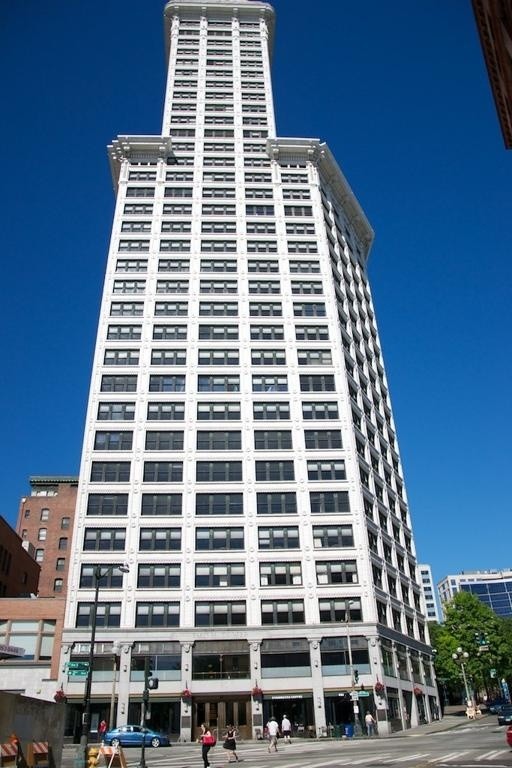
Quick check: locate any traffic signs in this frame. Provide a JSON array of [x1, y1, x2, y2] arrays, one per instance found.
[[358, 690, 369, 697], [62, 670, 89, 678], [65, 662, 89, 669]]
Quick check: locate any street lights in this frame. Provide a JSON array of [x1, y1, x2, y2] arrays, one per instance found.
[[71, 560, 130, 768], [451, 646, 477, 719], [343, 597, 364, 739]]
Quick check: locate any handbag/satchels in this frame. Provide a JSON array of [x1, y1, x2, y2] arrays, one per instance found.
[[202, 735, 216, 746]]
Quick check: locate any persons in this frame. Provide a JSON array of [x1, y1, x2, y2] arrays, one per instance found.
[[267, 716, 278, 755], [222, 724, 244, 762], [98, 719, 107, 742], [198, 723, 216, 768], [434, 704, 439, 720], [281, 715, 292, 744], [365, 710, 376, 737]]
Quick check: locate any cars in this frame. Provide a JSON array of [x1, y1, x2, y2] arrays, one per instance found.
[[489, 698, 508, 714], [505, 723, 512, 749], [103, 724, 171, 749], [496, 703, 511, 726]]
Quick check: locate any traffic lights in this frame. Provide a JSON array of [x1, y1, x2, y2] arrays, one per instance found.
[[145, 656, 152, 676], [479, 632, 486, 645], [474, 632, 481, 645], [148, 678, 158, 690], [354, 670, 359, 682], [484, 632, 490, 645]]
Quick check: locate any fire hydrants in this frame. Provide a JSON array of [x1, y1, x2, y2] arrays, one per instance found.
[[87, 745, 100, 768]]
